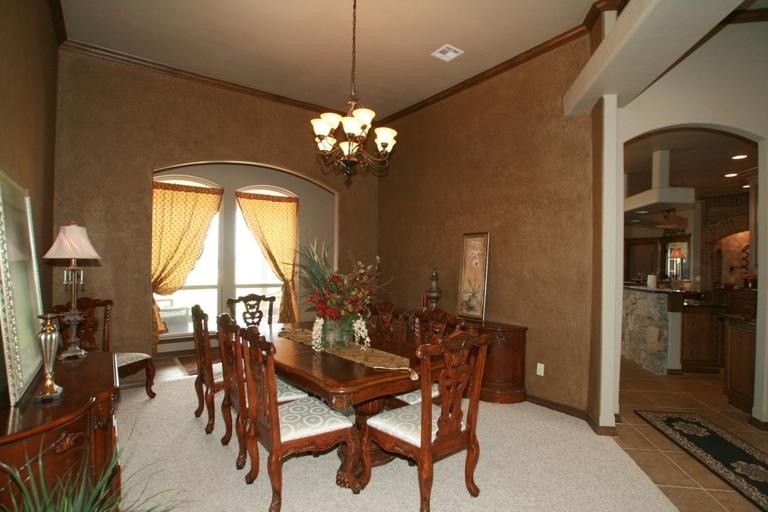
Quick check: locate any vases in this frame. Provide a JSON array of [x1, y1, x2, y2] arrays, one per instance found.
[[323, 318, 353, 348], [33, 313, 65, 404]]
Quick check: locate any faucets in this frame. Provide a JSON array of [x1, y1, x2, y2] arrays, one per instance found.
[[640, 276, 644, 284]]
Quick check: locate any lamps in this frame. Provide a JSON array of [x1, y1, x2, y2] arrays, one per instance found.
[[311, 1, 398, 177], [669, 248, 685, 280], [41, 218, 101, 358]]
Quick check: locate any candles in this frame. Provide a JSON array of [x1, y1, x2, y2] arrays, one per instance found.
[[422, 295, 428, 307]]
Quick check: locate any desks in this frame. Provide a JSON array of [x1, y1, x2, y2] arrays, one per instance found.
[[216, 321, 468, 489]]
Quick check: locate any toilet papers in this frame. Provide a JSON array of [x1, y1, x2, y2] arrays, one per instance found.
[[647, 275, 657, 288]]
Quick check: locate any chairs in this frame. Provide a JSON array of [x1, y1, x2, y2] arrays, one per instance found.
[[154, 299, 198, 334], [227, 294, 276, 325], [383, 308, 466, 467], [192, 304, 247, 434], [361, 300, 408, 337], [239, 326, 361, 512], [357, 329, 496, 511], [217, 313, 311, 471], [53, 297, 156, 399]]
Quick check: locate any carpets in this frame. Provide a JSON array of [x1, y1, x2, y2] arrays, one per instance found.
[[172, 351, 223, 376], [633, 409, 768, 512]]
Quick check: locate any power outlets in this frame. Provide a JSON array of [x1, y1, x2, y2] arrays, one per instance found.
[[536, 362, 545, 377]]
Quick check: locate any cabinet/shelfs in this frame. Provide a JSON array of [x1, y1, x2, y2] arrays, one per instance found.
[[395, 307, 527, 404], [0, 349, 122, 512], [726, 290, 756, 315]]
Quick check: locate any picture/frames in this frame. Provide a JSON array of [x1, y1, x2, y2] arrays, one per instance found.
[[456, 230, 490, 323], [0, 164, 44, 405]]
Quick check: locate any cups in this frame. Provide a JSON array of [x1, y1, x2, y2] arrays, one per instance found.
[[647, 274, 658, 289]]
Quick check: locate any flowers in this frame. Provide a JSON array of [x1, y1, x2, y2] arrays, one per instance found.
[[281, 237, 381, 353]]
